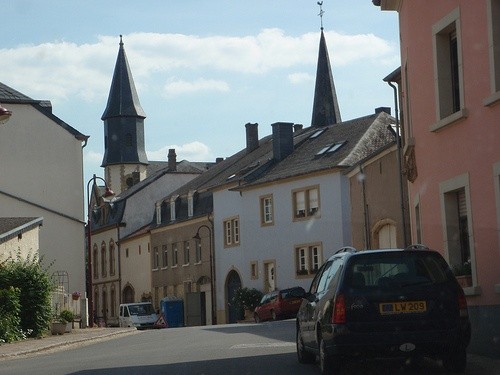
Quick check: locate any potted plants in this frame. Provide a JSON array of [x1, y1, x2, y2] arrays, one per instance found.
[[50, 310, 74, 336]]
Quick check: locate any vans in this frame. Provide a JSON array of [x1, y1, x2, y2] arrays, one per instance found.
[[253, 286, 308, 324], [118, 302, 159, 330]]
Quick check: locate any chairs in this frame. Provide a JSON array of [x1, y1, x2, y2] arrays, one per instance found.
[[352, 272, 367, 287]]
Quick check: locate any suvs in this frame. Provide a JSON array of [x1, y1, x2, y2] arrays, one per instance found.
[[288, 243, 471, 375]]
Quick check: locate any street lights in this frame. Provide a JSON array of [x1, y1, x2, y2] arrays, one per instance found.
[[87, 177, 116, 327], [192, 225, 215, 326]]
